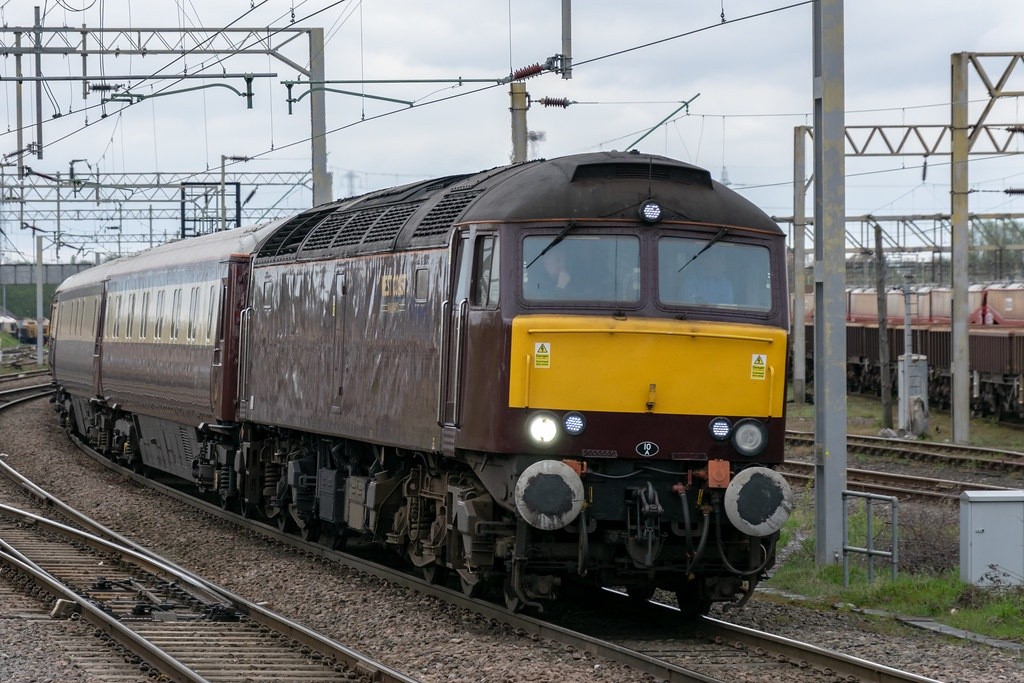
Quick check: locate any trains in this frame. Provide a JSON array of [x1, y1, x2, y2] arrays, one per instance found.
[[47, 151, 790, 625]]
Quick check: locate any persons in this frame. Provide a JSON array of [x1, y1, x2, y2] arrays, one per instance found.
[[535, 247, 580, 299]]
